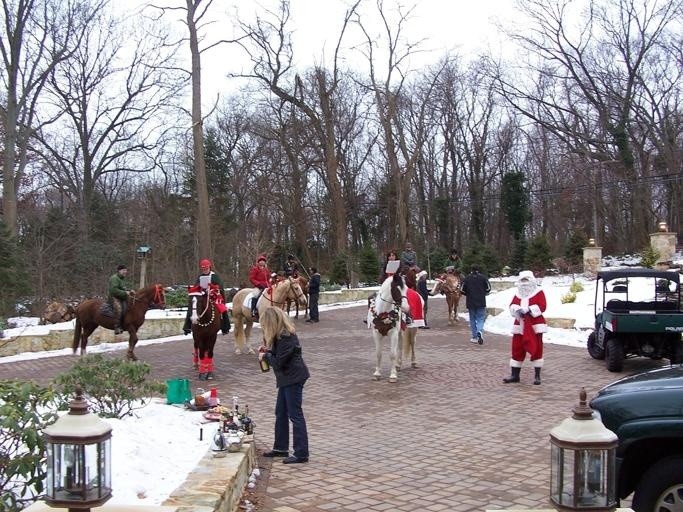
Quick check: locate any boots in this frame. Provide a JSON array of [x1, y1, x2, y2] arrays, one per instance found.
[[114, 325, 123, 335], [534, 366, 542, 385], [503, 366, 521, 383]]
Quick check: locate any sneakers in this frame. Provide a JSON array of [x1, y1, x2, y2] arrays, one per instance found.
[[477, 331, 484, 344], [470, 339, 478, 343]]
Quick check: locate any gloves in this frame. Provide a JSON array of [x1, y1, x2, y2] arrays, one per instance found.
[[519, 307, 529, 314]]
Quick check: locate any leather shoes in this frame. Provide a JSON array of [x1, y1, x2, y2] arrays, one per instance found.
[[283, 456, 308, 464], [263, 450, 289, 457]]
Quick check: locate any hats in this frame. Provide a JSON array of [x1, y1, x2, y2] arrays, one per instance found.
[[118, 264, 127, 270], [199, 260, 211, 270], [309, 267, 317, 270], [419, 270, 428, 276], [288, 255, 294, 259], [406, 243, 413, 248], [471, 264, 479, 271], [515, 271, 536, 298], [258, 256, 266, 262]]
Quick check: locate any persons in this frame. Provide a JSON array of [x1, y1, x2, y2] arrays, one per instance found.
[[440, 249, 466, 294], [400, 242, 418, 265], [183, 260, 231, 331], [378, 250, 404, 284], [243, 255, 281, 317], [107, 265, 135, 334], [460, 262, 491, 344], [415, 270, 431, 330], [258, 306, 311, 465], [503, 271, 548, 385], [283, 254, 300, 278], [304, 267, 320, 324]]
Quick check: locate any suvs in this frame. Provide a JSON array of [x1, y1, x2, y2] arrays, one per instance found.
[[589, 364, 683, 512]]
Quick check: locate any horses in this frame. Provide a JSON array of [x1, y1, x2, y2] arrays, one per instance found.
[[440, 269, 461, 325], [401, 265, 420, 289], [232, 276, 307, 354], [273, 277, 308, 320], [188, 285, 221, 381], [366, 269, 425, 382], [71, 282, 165, 360]]
